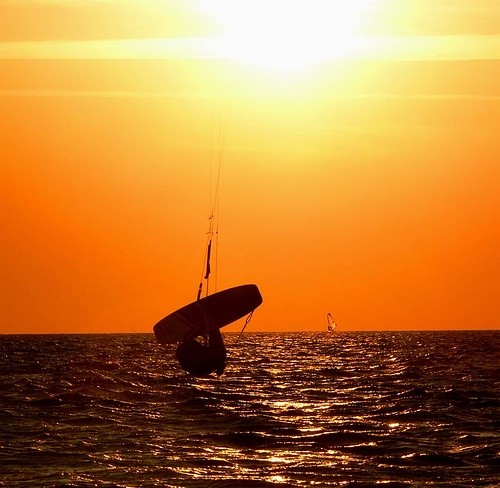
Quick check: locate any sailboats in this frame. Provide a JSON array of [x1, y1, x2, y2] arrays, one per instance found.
[[327, 313, 337, 332]]
